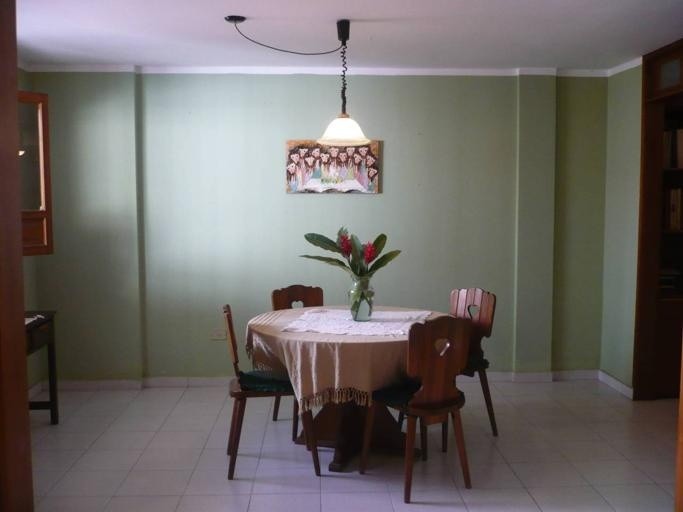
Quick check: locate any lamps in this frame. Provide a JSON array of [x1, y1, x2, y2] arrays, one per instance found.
[[222, 303, 322, 479], [397, 288, 497, 453], [358, 315, 473, 504], [270, 284, 323, 440]]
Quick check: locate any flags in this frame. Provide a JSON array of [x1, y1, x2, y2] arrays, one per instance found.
[[347, 280, 377, 322]]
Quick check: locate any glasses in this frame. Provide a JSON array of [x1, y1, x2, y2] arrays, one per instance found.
[[313, 19, 371, 148]]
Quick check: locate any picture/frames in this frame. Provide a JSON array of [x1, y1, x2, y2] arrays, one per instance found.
[[25, 310, 61, 425], [246, 305, 483, 472]]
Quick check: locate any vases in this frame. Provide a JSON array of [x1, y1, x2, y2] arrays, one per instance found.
[[300, 226, 402, 320]]
